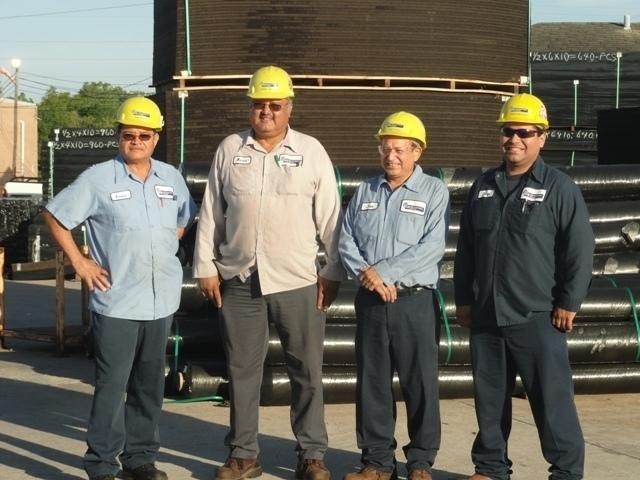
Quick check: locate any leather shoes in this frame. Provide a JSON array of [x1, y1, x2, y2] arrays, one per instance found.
[[343, 464, 397, 480], [215, 457, 264, 480], [122, 463, 168, 480], [89, 474, 116, 480], [407, 468, 433, 480], [467, 472, 494, 480], [295, 457, 333, 480]]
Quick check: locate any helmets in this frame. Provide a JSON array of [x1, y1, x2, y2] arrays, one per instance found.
[[115, 95, 165, 131], [246, 64, 294, 100], [496, 93, 551, 128], [374, 111, 427, 149]]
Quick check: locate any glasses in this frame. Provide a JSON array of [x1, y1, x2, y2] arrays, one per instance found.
[[119, 133, 153, 141], [252, 102, 287, 111], [501, 129, 542, 138]]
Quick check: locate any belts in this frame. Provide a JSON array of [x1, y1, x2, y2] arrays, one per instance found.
[[397, 284, 422, 300]]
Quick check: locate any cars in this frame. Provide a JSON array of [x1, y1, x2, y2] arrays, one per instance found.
[[3, 177, 44, 199]]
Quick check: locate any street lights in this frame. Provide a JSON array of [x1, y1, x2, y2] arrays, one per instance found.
[[8, 56, 23, 181]]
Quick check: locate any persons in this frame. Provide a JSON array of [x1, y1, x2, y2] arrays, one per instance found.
[[40, 94, 198, 480], [340, 109, 451, 480], [451, 93, 596, 480], [191, 64, 344, 480]]
